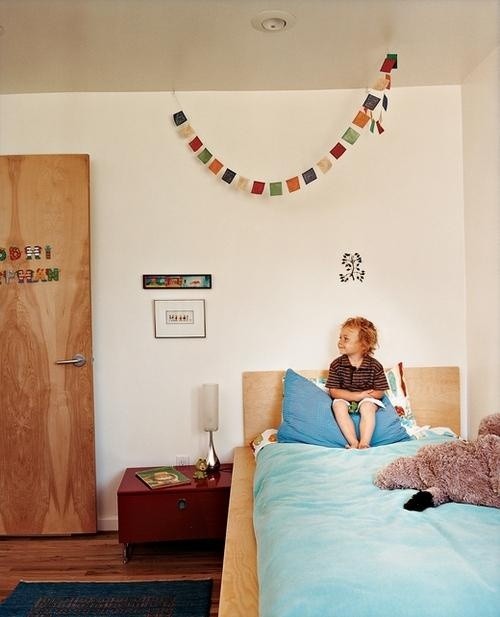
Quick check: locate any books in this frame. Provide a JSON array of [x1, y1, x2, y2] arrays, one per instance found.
[[134, 465, 192, 490]]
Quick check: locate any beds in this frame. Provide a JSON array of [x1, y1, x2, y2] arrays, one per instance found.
[[217, 366, 499, 616]]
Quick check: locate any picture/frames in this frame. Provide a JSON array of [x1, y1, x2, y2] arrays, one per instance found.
[[143, 273, 212, 338]]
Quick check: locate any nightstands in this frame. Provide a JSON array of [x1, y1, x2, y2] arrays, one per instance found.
[[116, 462, 233, 564]]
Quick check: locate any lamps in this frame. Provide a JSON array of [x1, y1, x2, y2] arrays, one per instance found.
[[199, 382, 220, 471]]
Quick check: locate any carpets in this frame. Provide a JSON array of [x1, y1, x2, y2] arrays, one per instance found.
[[2, 579, 214, 616]]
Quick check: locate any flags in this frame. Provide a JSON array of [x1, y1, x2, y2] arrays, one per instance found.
[[173, 52, 399, 197]]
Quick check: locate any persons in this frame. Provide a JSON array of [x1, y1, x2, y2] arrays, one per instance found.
[[323, 315, 390, 448], [147, 469, 178, 482]]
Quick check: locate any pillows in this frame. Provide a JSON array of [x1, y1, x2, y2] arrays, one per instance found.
[[275, 362, 419, 447]]
